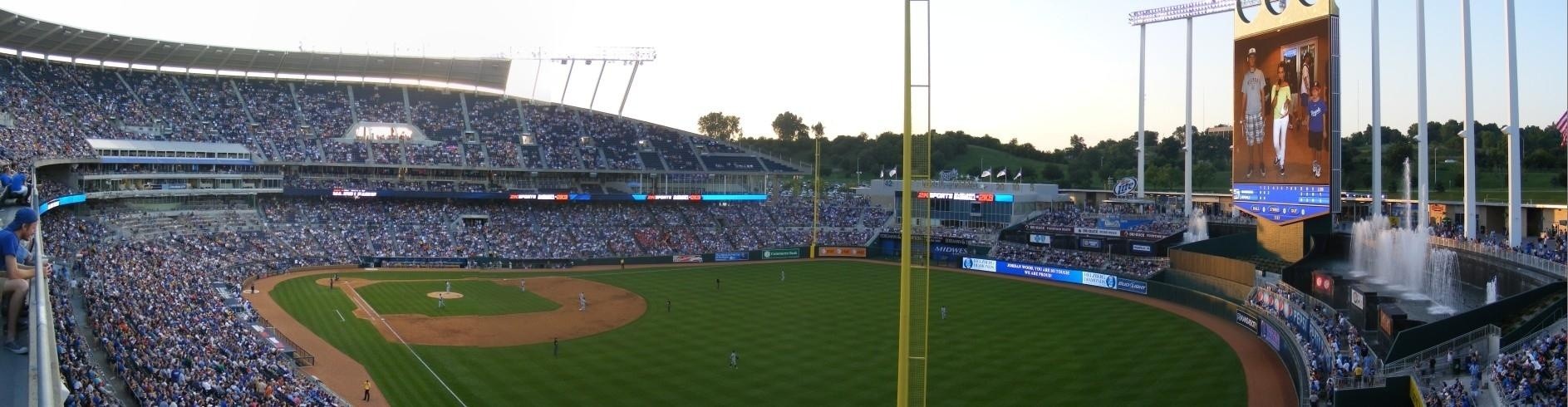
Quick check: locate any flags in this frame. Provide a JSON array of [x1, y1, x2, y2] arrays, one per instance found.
[[1557, 112, 1568, 146], [981, 169, 1021, 180], [880, 167, 896, 177]]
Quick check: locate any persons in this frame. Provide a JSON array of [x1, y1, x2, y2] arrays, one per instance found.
[[1272, 64, 1291, 175], [521, 279, 525, 292], [741, 181, 1232, 280], [717, 279, 720, 287], [345, 84, 741, 270], [578, 292, 585, 311], [1288, 60, 1298, 129], [1248, 202, 1568, 406], [554, 337, 558, 355], [1300, 55, 1313, 126], [363, 379, 370, 401], [446, 281, 450, 292], [438, 293, 445, 308], [1240, 48, 1266, 179], [1307, 82, 1326, 177], [666, 300, 671, 312], [941, 306, 946, 319], [730, 351, 738, 368], [1, 53, 345, 407]]
